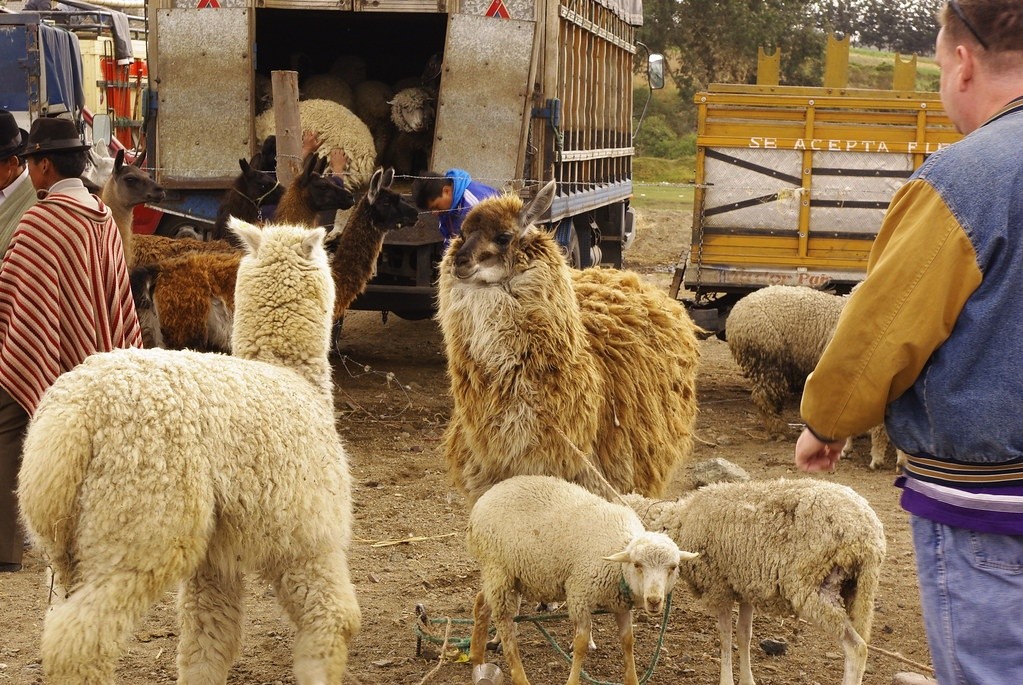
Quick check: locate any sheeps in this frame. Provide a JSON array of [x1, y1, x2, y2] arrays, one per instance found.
[[465, 476, 700, 685], [129, 153, 419, 356], [253, 99, 377, 194], [102, 149, 166, 269], [255, 74, 305, 116], [725, 284, 850, 441], [387, 77, 439, 174], [612, 477, 886, 685], [302, 75, 393, 162], [88, 139, 126, 188], [842, 281, 908, 474]]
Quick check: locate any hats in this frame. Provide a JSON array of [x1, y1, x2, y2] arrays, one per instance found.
[[0, 108, 29, 160], [18, 118, 92, 156]]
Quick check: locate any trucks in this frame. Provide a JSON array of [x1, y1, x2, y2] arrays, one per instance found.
[[0, 0, 148, 164], [137, 1, 665, 321]]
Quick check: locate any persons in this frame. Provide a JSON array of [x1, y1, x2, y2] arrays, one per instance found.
[[792, 0, 1022, 685], [0, 117, 142, 427], [411, 169, 499, 239], [0, 104, 37, 573]]
